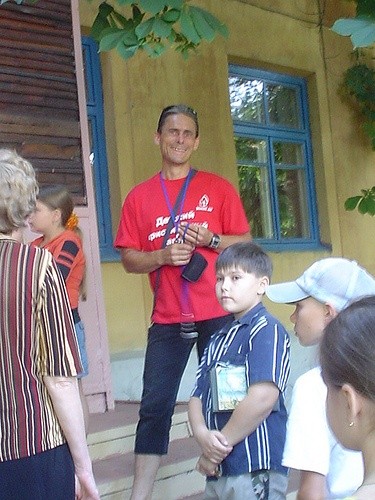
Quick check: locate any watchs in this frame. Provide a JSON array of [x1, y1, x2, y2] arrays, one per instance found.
[[208, 233, 221, 250]]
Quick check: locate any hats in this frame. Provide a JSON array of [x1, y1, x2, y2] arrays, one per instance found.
[[263, 257, 375, 313]]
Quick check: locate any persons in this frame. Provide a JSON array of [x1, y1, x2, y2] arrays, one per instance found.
[[318, 295, 375, 500], [265, 255, 375, 500], [113, 105, 252, 500], [0, 146, 101, 500], [26, 185, 89, 442], [187, 241, 291, 499]]
